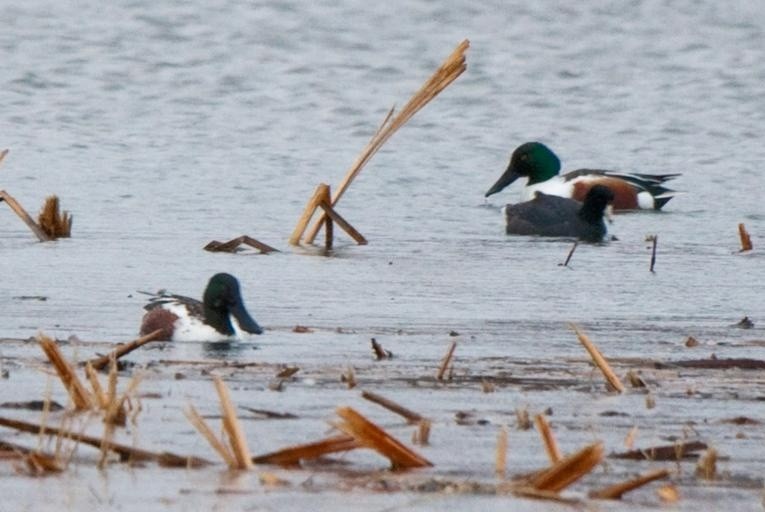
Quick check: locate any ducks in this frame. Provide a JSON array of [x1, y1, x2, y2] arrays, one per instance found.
[[500, 186, 614, 241], [485, 142, 688, 212], [140, 274, 263, 343]]
